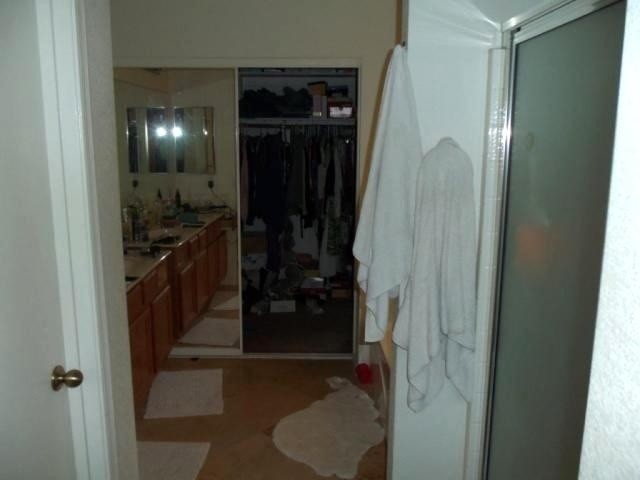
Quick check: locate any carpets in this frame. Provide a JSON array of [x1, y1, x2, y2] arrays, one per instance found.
[[178, 296, 243, 347], [132, 367, 246, 480]]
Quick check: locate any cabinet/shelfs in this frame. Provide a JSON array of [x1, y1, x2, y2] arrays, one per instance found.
[[128, 254, 172, 374], [233, 67, 357, 359], [172, 219, 227, 345]]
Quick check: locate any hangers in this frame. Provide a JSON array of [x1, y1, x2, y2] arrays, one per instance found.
[[240, 120, 355, 144]]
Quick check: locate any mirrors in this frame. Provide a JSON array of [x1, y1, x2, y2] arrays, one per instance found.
[[114, 59, 244, 361]]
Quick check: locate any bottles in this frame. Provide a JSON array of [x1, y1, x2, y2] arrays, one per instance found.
[[175, 189, 181, 209]]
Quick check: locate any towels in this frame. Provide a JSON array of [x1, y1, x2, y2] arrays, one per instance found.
[[390, 136, 479, 417], [352, 44, 425, 347]]
